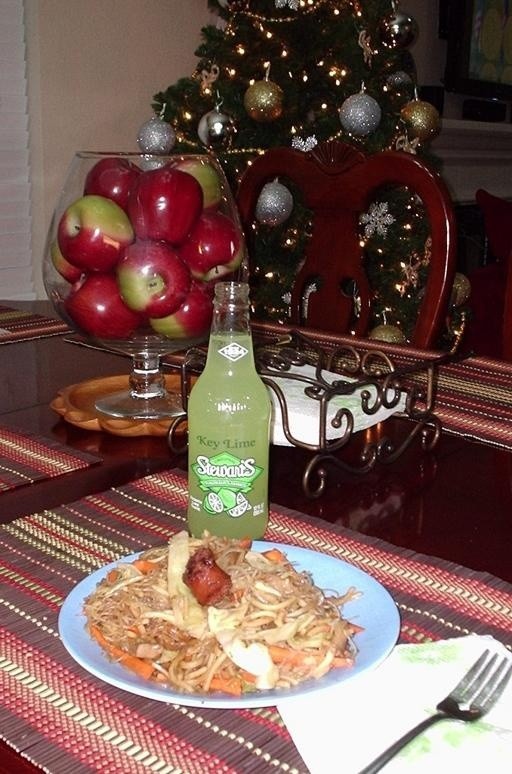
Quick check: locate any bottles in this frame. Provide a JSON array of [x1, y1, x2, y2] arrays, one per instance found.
[[188, 281, 271, 543]]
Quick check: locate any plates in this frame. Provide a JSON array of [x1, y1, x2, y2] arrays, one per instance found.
[[56, 538, 402, 709]]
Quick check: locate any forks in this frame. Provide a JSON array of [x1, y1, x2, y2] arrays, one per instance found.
[[361, 649, 512, 768]]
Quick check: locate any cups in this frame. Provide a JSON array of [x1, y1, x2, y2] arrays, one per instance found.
[[41, 147, 248, 422]]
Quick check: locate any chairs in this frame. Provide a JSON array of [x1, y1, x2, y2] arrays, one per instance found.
[[237, 141, 456, 346]]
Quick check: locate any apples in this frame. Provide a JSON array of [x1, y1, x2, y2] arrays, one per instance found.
[[63, 271, 143, 342], [83, 158, 145, 213], [57, 195, 135, 274], [50, 236, 82, 285], [165, 157, 222, 216], [149, 280, 212, 340], [176, 212, 245, 284], [128, 168, 204, 248], [116, 239, 191, 319]]
